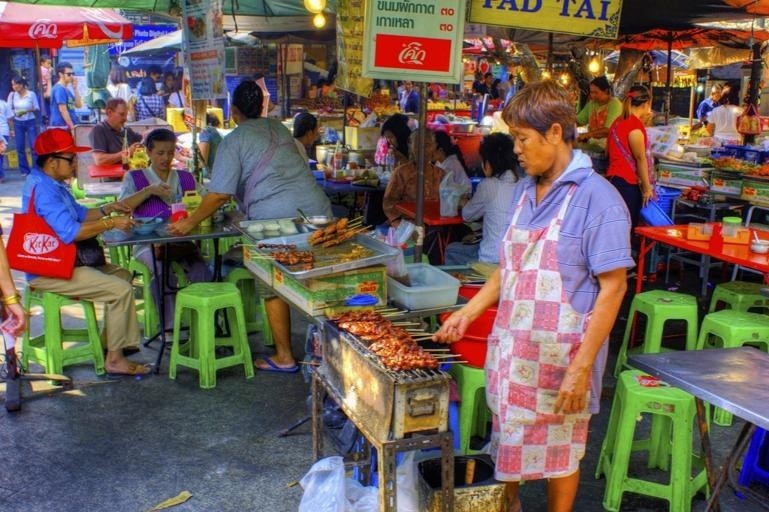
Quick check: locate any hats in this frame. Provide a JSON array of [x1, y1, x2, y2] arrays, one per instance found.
[[34, 128, 92, 155]]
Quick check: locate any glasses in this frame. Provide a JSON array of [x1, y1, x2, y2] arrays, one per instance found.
[[51, 154, 76, 165], [63, 73, 76, 77]]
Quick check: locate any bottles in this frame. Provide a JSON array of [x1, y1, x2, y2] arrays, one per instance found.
[[120, 136, 131, 170], [171, 195, 188, 235], [333, 139, 343, 170]]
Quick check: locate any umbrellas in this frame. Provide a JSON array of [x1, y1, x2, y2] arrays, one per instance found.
[[570, 10, 769, 126], [119, 25, 262, 57], [0, 0, 134, 123]]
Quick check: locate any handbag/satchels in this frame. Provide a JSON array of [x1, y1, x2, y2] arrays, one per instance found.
[[639, 172, 658, 202], [5, 213, 77, 279]]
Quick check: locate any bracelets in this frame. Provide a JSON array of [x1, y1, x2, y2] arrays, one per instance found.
[[0, 288, 24, 306]]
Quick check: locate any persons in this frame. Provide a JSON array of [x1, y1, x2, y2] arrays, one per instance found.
[[0, 223, 30, 338], [120, 128, 216, 345], [165, 78, 334, 375], [21, 129, 153, 377], [430, 80, 640, 510], [1, 53, 768, 280]]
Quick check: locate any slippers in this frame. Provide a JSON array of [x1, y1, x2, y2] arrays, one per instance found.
[[252, 355, 298, 373], [105, 362, 151, 378]]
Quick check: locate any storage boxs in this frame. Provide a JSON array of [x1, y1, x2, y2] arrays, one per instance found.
[[309, 125, 381, 169], [645, 118, 769, 206]]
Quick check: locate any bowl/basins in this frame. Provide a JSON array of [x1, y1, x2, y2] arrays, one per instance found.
[[750, 240, 769, 254], [130, 217, 163, 235], [449, 121, 479, 134]]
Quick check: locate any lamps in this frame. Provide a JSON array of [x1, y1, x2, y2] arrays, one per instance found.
[[587, 39, 601, 73]]
